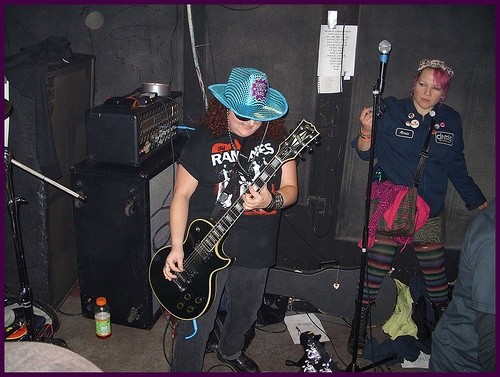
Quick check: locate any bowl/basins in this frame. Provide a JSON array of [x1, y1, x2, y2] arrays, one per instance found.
[[143, 83, 170, 96]]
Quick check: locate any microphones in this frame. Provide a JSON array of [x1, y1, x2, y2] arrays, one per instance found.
[[378, 39, 391, 95]]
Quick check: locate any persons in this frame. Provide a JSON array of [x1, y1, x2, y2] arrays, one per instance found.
[[163, 69, 298, 372], [430, 197, 496, 373], [348, 59, 488, 356]]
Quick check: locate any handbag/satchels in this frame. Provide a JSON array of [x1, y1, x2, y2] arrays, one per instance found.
[[372, 187, 417, 236]]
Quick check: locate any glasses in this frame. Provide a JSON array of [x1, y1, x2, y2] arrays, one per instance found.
[[234, 112, 251, 121]]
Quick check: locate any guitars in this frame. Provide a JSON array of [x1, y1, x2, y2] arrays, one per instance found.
[[147, 118, 323, 321]]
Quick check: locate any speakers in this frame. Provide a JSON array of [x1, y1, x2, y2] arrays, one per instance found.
[[70, 135, 188, 332], [4, 52, 95, 313]]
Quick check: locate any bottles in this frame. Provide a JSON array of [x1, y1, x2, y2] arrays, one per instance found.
[[94, 297, 111, 339]]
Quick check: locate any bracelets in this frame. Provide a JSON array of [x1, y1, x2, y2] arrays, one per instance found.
[[358, 127, 371, 139], [263, 193, 276, 213], [273, 191, 284, 210], [360, 137, 371, 144]]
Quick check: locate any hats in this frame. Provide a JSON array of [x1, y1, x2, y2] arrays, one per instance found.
[[207, 67, 288, 121]]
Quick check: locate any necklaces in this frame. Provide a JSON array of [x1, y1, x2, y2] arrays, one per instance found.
[[226, 108, 269, 174]]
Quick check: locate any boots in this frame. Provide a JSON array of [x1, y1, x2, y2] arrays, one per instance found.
[[347, 301, 376, 358], [432, 301, 449, 327]]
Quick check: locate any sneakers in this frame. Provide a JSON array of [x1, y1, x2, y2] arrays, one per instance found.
[[216, 350, 261, 372]]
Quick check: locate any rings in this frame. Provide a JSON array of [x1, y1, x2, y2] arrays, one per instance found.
[[165, 265, 170, 268]]
